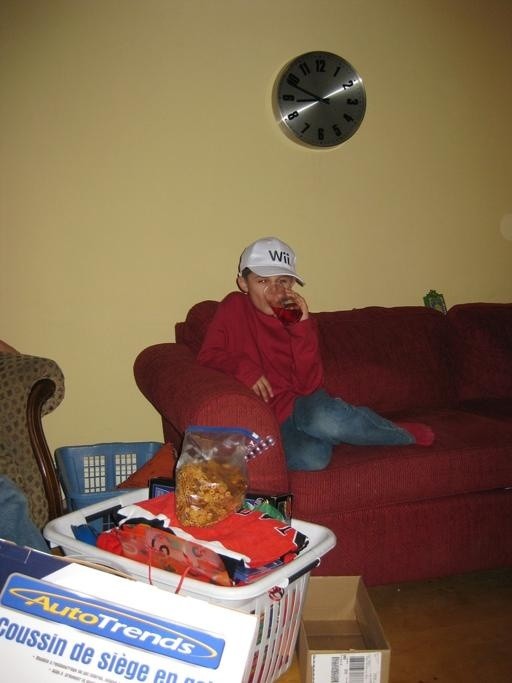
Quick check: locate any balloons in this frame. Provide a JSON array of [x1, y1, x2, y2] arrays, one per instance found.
[[276, 49, 367, 148]]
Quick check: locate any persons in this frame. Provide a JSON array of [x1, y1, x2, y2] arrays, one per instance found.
[[192, 237, 439, 470]]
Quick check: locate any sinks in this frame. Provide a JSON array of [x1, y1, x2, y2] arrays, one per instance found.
[[132, 299, 509, 583]]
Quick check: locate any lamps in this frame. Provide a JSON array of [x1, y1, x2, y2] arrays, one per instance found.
[[1, 337, 68, 558]]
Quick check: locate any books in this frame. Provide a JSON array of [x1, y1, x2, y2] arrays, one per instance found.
[[0, 562, 261, 683], [298, 576, 392, 683]]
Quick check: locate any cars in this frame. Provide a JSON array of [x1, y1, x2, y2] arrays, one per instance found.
[[264, 284, 303, 326]]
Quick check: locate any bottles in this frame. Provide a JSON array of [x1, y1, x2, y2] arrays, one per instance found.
[[239, 237, 305, 287]]
